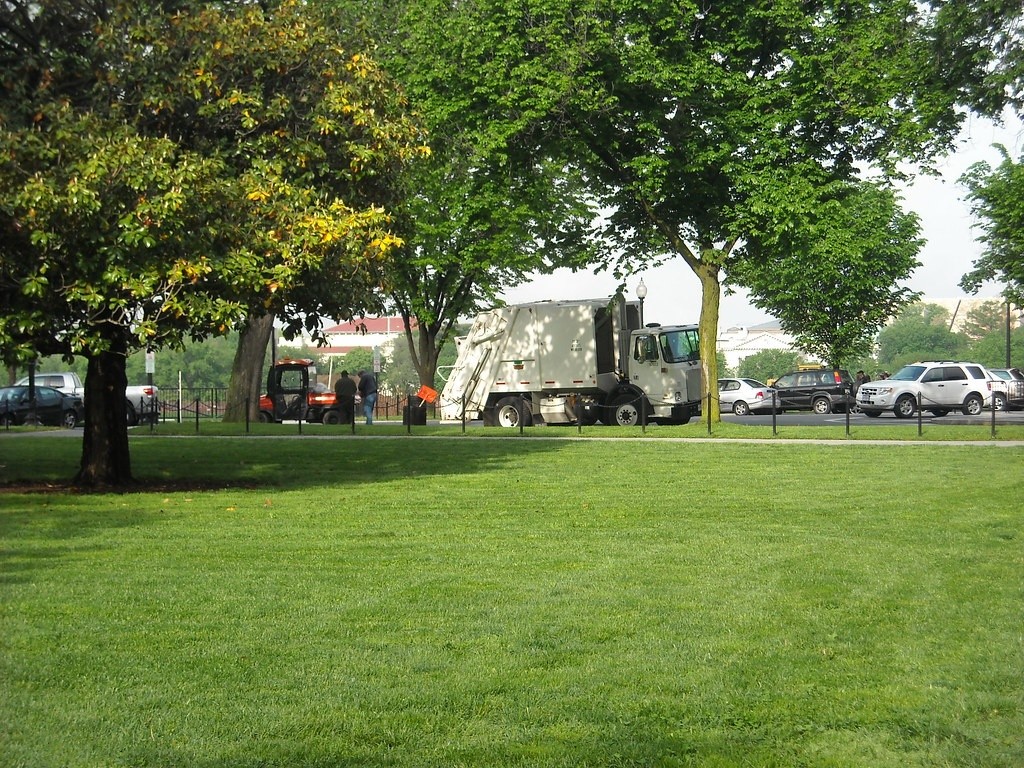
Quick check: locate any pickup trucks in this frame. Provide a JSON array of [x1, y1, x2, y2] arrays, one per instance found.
[[0, 372, 160, 426]]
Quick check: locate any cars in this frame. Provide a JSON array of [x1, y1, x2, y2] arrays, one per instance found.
[[0, 384, 85, 427], [696, 377, 782, 416]]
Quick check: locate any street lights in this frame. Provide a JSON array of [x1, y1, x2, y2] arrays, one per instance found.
[[1003, 281, 1015, 369], [634, 276, 649, 330]]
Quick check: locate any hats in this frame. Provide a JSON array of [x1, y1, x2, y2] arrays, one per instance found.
[[340, 370, 349, 374], [857, 370, 864, 374]]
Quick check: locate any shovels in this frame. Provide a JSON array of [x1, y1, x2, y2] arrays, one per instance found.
[[417, 385, 437, 407]]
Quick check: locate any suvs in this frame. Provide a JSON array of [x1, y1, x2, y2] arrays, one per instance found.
[[767, 369, 856, 415], [983, 368, 1024, 411], [855, 360, 994, 419]]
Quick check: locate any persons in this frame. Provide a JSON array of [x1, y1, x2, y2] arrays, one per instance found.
[[358, 369, 377, 426], [334, 370, 357, 425], [849, 370, 892, 415]]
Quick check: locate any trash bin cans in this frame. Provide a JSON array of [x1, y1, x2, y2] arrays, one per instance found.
[[412, 407, 426, 425], [403, 406, 413, 425], [407, 395, 426, 407]]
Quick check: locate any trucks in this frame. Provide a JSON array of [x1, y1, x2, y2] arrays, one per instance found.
[[438, 298, 699, 428]]
